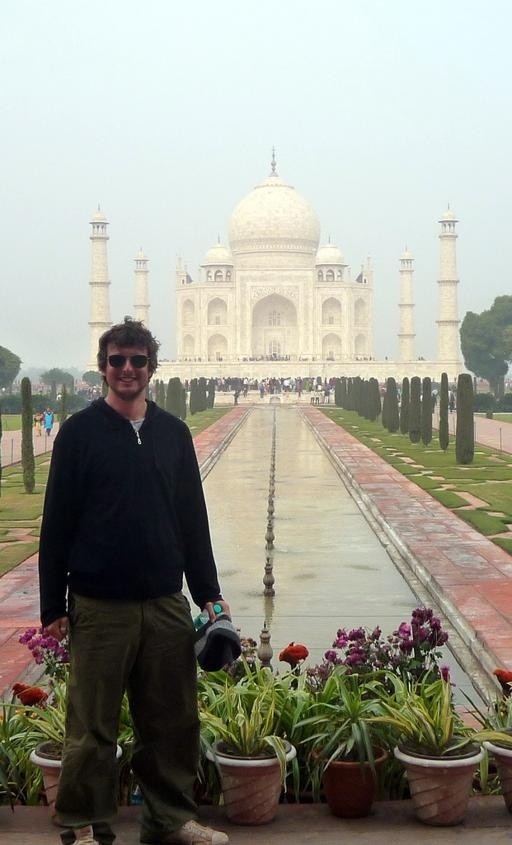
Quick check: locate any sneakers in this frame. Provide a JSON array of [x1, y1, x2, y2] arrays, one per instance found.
[[71, 824, 100, 845], [141, 818, 229, 845]]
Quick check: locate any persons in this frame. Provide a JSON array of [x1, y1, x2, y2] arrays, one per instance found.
[[35, 407, 54, 437], [37, 314, 237, 845], [202, 373, 458, 412]]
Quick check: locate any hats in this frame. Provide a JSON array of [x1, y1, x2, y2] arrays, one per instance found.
[[193, 612, 243, 672]]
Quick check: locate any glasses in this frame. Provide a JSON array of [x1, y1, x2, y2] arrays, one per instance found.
[[106, 353, 152, 369]]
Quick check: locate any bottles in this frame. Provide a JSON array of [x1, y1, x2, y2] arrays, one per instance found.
[[193, 604, 222, 630]]
